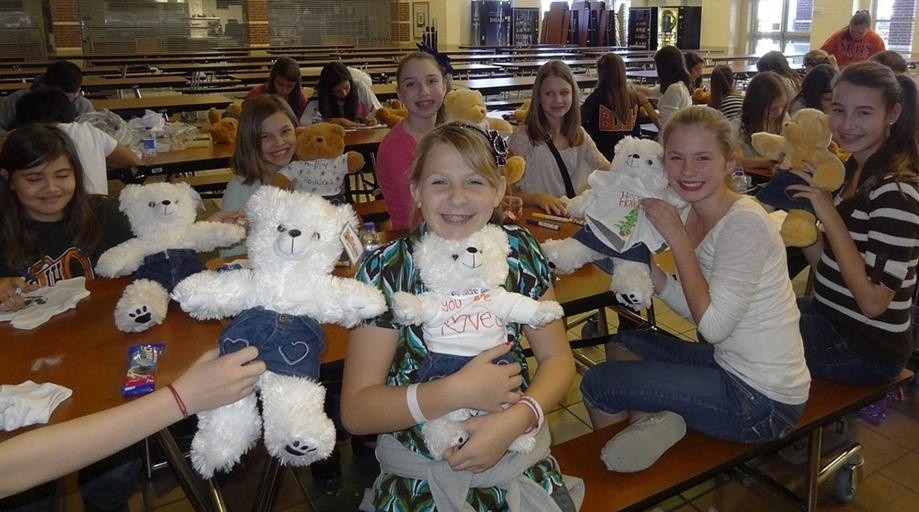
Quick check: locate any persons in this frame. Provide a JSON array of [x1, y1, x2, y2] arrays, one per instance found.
[[220, 93, 345, 257], [1, 346, 266, 510], [242, 58, 307, 125], [0, 61, 94, 131], [340, 120, 585, 510], [377, 51, 523, 232], [0, 123, 247, 311], [17, 89, 140, 196], [508, 6, 919, 472], [298, 62, 383, 171]]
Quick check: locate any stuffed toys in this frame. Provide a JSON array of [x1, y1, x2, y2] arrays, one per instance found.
[[208, 99, 245, 144], [375, 99, 409, 127], [392, 224, 564, 461], [443, 89, 527, 186], [94, 182, 246, 334], [272, 122, 366, 207], [173, 181, 388, 482]]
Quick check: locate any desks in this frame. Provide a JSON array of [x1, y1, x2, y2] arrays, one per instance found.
[[0, 206, 675, 511]]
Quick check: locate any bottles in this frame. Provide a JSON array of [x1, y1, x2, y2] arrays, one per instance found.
[[144, 126, 158, 161], [311, 107, 322, 125], [732, 167, 744, 180], [732, 170, 746, 193], [361, 222, 380, 260]]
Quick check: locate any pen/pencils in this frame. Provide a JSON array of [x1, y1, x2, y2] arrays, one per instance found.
[[527, 212, 574, 231], [15, 266, 28, 295]]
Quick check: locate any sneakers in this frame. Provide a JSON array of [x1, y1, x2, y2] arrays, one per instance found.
[[311, 447, 339, 496], [599, 410, 687, 474]]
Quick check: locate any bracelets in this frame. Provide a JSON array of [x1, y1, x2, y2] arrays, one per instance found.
[[166, 383, 188, 421], [406, 382, 428, 427], [517, 395, 546, 435]]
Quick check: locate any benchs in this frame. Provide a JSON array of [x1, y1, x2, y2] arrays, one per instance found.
[[551, 366, 913, 512]]
